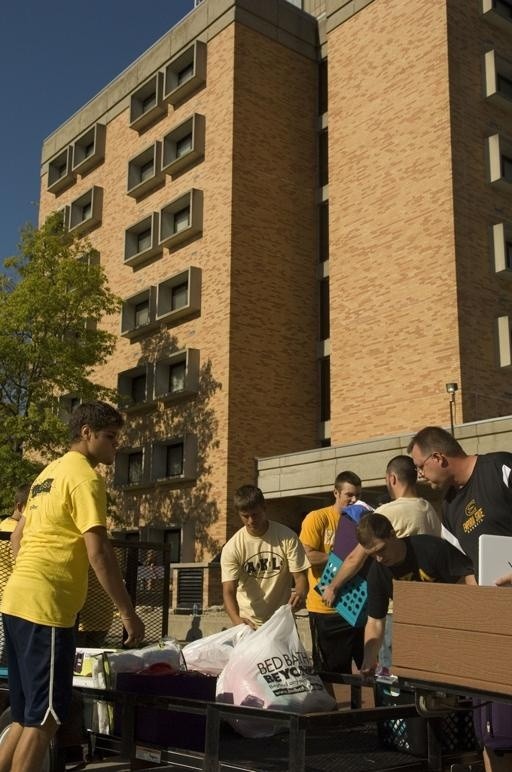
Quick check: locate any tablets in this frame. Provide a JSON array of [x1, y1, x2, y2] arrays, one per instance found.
[[477, 534, 512, 586]]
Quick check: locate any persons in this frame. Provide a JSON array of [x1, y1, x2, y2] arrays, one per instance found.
[[217, 420, 511, 772], [0, 400, 145, 771]]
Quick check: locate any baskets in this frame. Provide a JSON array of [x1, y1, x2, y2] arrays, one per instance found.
[[373, 685, 479, 757], [318, 552, 368, 628]]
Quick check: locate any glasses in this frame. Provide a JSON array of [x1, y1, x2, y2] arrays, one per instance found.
[[414, 455, 432, 478]]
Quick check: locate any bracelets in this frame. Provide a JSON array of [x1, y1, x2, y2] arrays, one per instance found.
[[117, 610, 137, 621]]
